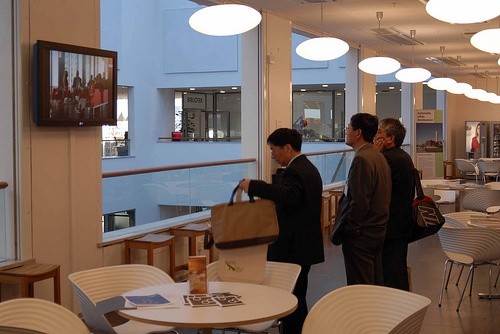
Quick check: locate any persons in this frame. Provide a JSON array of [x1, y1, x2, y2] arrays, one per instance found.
[[373, 117, 416, 291], [472, 123, 481, 152], [331, 113, 393, 286], [241, 127, 325, 334], [62, 69, 102, 91]]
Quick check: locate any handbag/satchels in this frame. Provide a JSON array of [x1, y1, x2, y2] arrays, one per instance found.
[[406, 168, 445, 243], [203, 179, 280, 251]]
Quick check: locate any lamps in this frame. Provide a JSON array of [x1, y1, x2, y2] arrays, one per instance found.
[[189, 0, 500, 105]]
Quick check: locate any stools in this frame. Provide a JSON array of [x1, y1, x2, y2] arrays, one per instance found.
[[329, 188, 343, 236], [170, 223, 213, 273], [125, 233, 175, 281], [0, 262, 61, 307], [321, 194, 332, 242]]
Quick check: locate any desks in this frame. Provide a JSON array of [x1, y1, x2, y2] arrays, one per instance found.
[[466, 215, 500, 300], [117, 281, 298, 334]]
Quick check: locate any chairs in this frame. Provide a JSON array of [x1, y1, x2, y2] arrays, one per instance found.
[[0, 156, 500, 334]]
